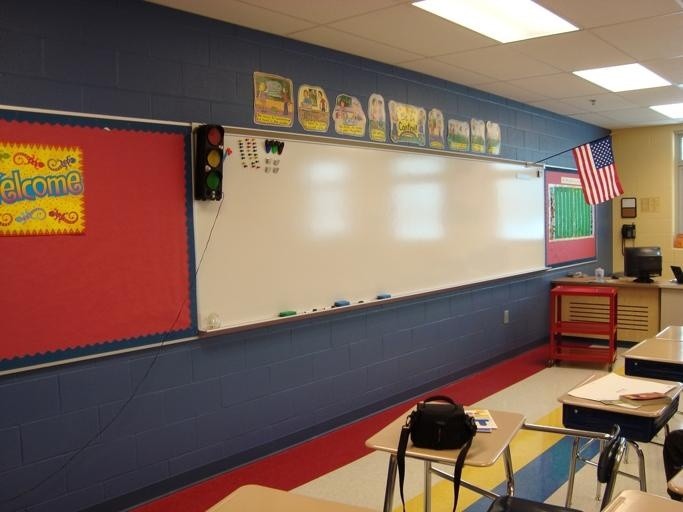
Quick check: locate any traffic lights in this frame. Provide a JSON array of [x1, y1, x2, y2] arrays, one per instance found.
[[194, 123, 223, 201]]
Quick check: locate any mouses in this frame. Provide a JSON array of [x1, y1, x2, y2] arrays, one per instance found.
[[612, 275, 619, 279]]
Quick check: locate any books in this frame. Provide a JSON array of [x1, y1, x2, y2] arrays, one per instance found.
[[463, 409, 498, 434], [620, 392, 673, 406]]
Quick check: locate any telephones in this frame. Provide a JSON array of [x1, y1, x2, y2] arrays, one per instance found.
[[622, 224, 636, 239], [622, 225, 635, 239]]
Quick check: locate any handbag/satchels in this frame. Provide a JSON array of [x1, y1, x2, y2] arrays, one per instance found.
[[411, 396, 477, 448]]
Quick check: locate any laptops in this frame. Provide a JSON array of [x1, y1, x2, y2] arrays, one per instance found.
[[671, 266, 683, 284]]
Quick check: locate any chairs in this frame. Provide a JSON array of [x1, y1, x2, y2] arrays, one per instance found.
[[665, 470, 682, 502], [484, 425, 626, 512]]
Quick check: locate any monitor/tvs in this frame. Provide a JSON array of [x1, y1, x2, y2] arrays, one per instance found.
[[624, 246, 662, 283]]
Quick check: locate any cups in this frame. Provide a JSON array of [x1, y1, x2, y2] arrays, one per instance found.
[[595, 268, 604, 282]]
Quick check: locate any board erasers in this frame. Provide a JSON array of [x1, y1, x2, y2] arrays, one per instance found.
[[278, 311, 296, 316], [375, 293, 390, 299], [334, 301, 350, 306]]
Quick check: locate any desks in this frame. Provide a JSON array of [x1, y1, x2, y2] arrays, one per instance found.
[[599, 486, 682, 511], [206, 481, 389, 512], [558, 370, 681, 508], [369, 398, 526, 512], [621, 322, 683, 448]]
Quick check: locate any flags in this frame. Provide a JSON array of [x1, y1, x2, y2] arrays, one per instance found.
[[573, 135, 624, 206]]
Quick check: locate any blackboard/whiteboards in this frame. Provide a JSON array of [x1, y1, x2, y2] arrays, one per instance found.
[[198, 129, 542, 328]]
